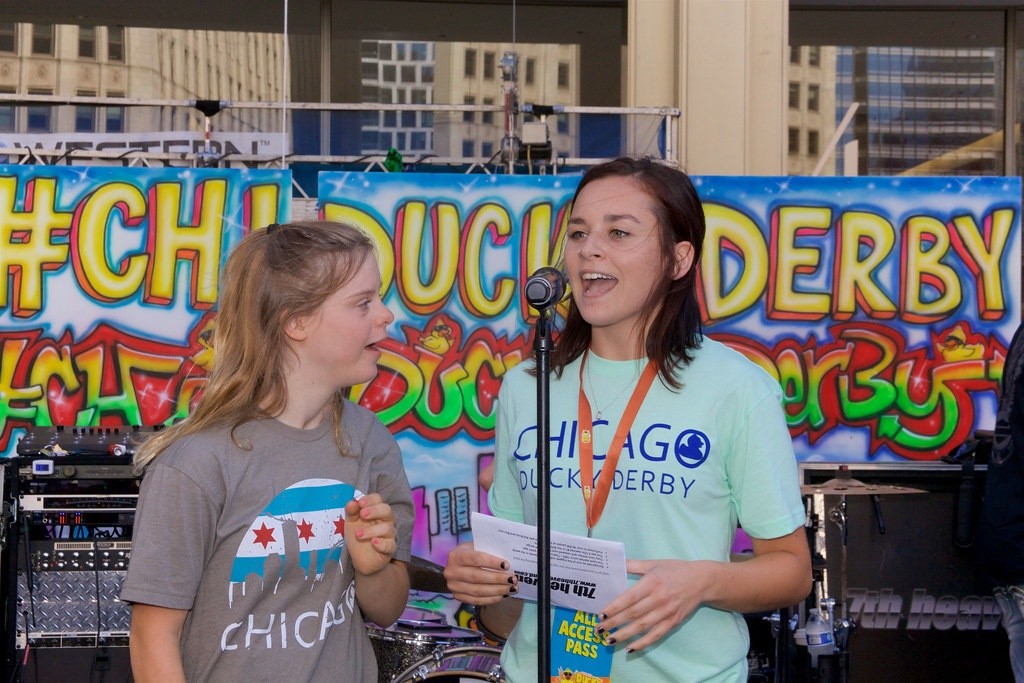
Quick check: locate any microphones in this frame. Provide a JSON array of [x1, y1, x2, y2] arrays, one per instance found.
[[524, 267, 566, 307]]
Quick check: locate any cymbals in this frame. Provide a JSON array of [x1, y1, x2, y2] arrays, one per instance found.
[[798, 480, 929, 495]]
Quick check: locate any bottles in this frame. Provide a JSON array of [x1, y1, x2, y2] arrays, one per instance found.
[[805, 608, 835, 668]]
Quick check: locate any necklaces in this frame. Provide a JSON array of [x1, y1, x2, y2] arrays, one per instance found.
[[584, 353, 645, 420]]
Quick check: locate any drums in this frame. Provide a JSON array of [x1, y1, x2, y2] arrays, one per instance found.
[[354, 607, 525, 682]]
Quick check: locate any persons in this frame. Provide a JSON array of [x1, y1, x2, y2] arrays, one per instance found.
[[974, 323, 1024, 681], [120, 218, 415, 683], [443, 156, 814, 682]]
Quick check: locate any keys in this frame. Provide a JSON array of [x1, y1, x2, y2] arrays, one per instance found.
[[52, 443, 71, 456]]
